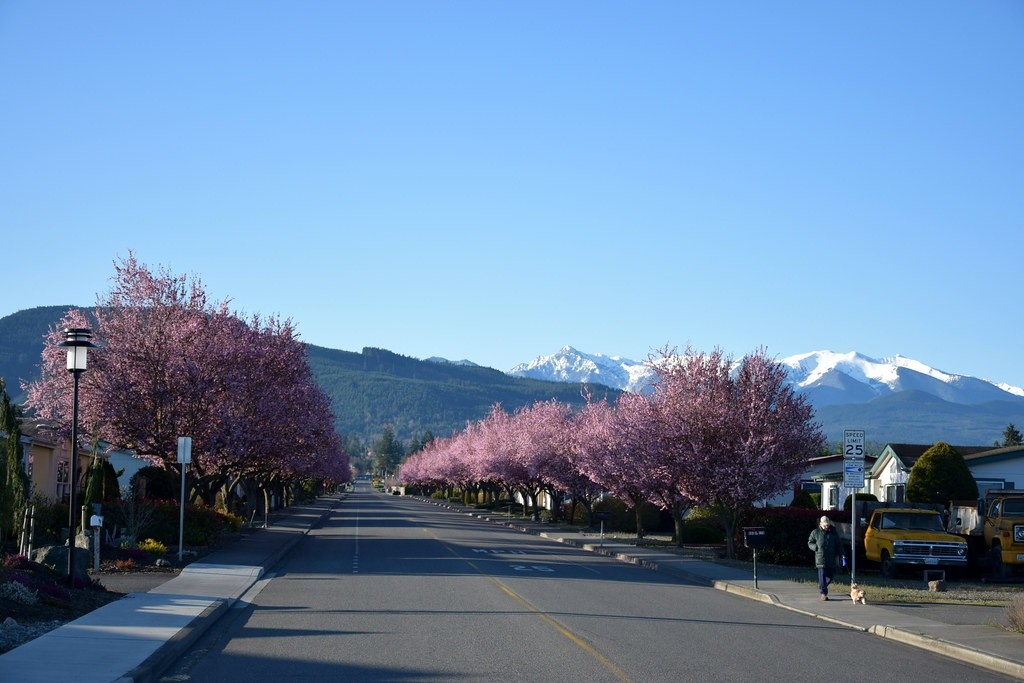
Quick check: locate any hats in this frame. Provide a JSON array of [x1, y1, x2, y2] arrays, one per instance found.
[[820, 516, 830, 525]]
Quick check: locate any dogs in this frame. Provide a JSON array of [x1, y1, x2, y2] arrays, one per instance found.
[[850, 583, 866, 606]]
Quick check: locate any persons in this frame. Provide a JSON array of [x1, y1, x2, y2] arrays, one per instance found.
[[808, 516, 843, 601], [943, 509, 952, 529]]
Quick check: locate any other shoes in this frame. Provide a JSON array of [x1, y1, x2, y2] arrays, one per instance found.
[[821, 594, 828, 600]]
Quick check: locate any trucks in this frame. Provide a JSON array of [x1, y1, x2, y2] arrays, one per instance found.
[[946, 487, 1024, 580], [826, 500, 970, 580]]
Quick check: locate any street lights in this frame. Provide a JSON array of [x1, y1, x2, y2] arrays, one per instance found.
[[56, 325, 99, 577]]
